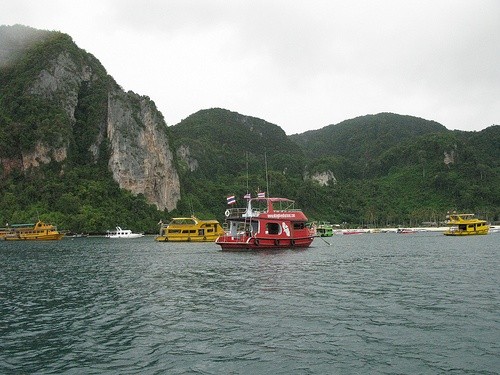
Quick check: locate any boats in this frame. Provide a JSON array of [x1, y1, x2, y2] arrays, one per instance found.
[[61, 230, 88, 238], [0, 210, 64, 241], [305, 219, 333, 238], [443, 211, 490, 236], [156, 199, 226, 242], [215, 151, 315, 251], [105, 225, 145, 239], [342, 228, 427, 234]]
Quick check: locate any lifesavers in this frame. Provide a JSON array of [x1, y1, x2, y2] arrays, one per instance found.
[[255, 239, 260, 245], [203, 237, 206, 240], [291, 240, 295, 245], [225, 210, 230, 217], [165, 238, 168, 241], [275, 240, 279, 246]]
